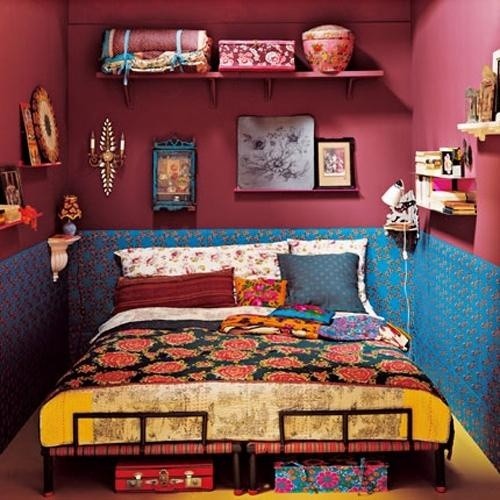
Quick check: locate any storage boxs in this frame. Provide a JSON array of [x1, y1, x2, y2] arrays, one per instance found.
[[273, 454, 387, 494], [218, 39, 296, 72]]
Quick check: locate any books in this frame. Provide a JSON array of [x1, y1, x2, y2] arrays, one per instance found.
[[416, 150, 477, 216]]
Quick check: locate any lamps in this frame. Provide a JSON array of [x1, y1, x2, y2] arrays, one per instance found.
[[380, 180, 410, 334], [58, 194, 82, 235]]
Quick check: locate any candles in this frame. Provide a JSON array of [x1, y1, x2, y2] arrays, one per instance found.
[[118, 129, 127, 151], [90, 130, 97, 149]]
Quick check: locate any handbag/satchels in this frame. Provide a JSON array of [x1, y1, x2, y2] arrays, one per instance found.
[[274, 462, 388, 493]]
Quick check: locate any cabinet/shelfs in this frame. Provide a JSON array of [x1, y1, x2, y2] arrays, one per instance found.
[[412, 170, 478, 219]]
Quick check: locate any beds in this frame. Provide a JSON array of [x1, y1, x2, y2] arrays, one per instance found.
[[39, 238, 455, 497]]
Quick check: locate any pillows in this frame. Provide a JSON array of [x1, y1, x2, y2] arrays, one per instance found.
[[288, 238, 377, 315], [114, 241, 288, 303], [276, 252, 368, 313], [235, 278, 287, 307], [113, 266, 237, 313]]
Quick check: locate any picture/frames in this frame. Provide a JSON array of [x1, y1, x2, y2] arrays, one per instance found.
[[314, 137, 356, 190]]
